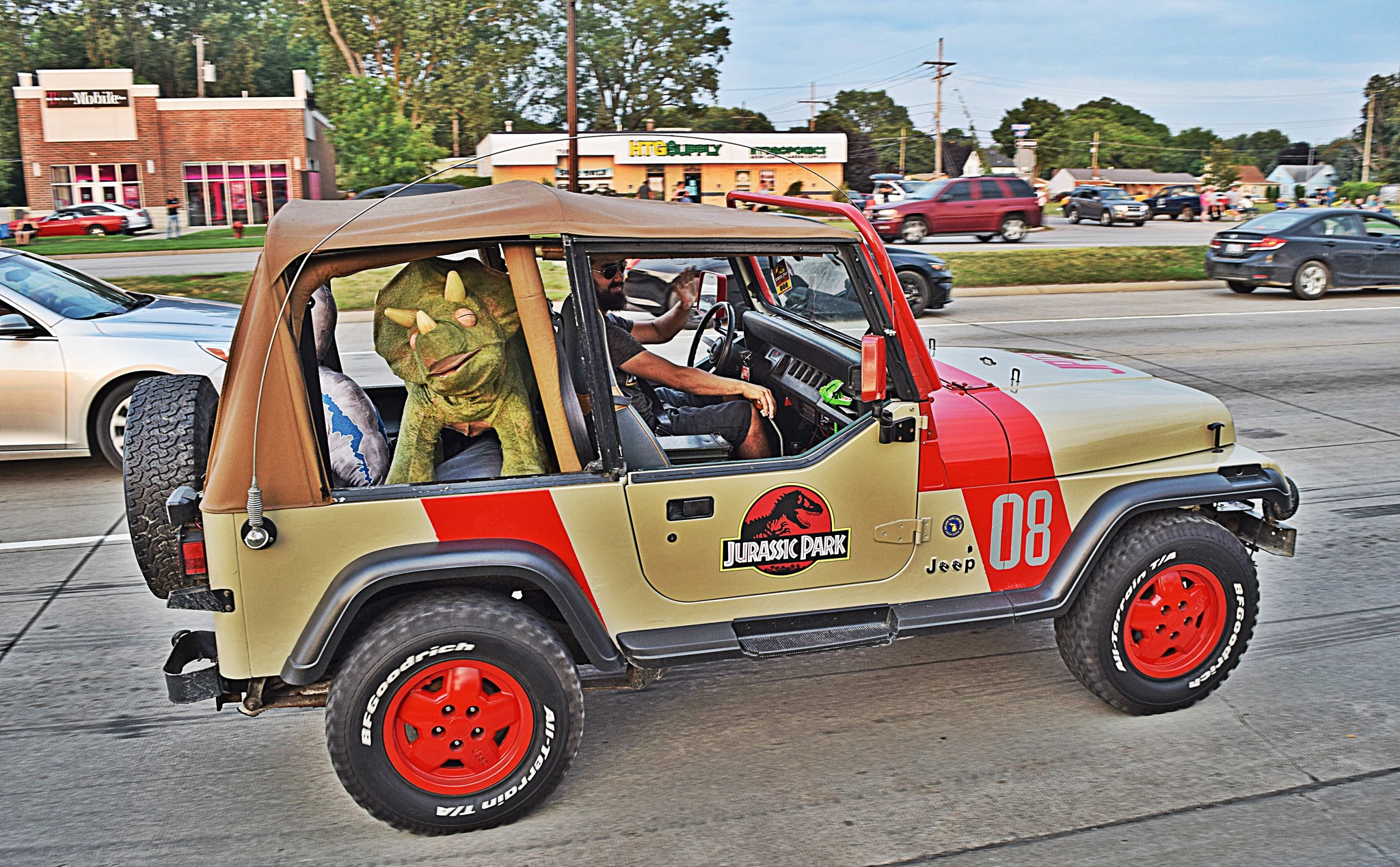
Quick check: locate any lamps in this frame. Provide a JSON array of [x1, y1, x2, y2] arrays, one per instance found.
[[146, 160, 155, 174], [32, 162, 43, 178]]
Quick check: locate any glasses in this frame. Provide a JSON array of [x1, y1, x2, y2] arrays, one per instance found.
[[592, 260, 626, 280]]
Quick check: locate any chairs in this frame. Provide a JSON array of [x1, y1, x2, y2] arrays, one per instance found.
[[1333, 225, 1345, 234], [8, 228, 39, 244], [560, 293, 675, 466]]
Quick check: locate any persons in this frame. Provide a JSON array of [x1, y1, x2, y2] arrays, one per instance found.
[[636, 180, 655, 200], [561, 259, 779, 461], [1275, 197, 1290, 210], [1198, 182, 1244, 222], [879, 179, 894, 202], [672, 181, 693, 203], [166, 191, 182, 239], [15, 213, 39, 246], [1340, 194, 1393, 218], [1297, 197, 1312, 209], [1034, 187, 1048, 212]]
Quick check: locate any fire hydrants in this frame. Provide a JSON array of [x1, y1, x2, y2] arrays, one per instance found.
[[232, 221, 243, 239]]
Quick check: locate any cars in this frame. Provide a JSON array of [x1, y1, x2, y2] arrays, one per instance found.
[[7, 208, 129, 238], [1203, 207, 1400, 301], [239, 189, 287, 210], [352, 183, 464, 200], [67, 202, 153, 235], [623, 244, 955, 330], [1055, 191, 1072, 202], [0, 246, 242, 473], [845, 171, 949, 212]]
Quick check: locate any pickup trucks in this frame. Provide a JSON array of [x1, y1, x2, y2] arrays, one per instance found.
[[1141, 184, 1230, 222]]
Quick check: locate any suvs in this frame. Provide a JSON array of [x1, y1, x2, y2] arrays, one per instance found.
[[120, 178, 1299, 839], [1062, 184, 1153, 228], [863, 173, 1046, 244]]
